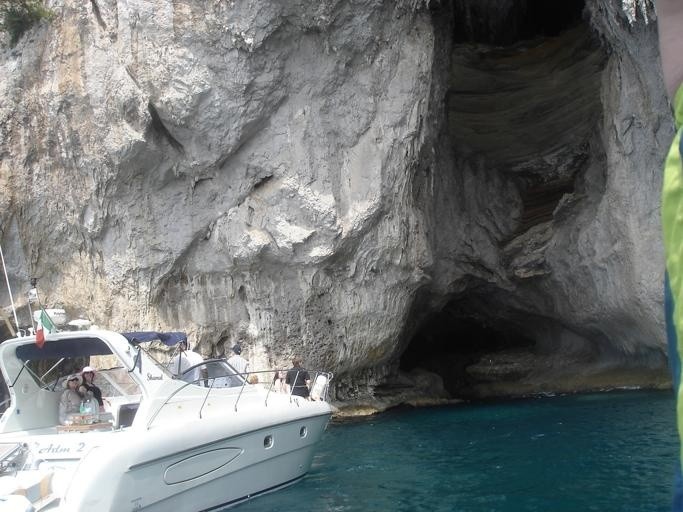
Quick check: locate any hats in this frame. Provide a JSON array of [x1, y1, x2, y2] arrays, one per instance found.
[[67, 374, 79, 382], [81, 366, 96, 377]]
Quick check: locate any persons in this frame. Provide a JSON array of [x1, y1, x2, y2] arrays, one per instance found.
[[249, 373, 257, 384], [272, 370, 286, 394], [286, 356, 313, 402], [56, 373, 84, 426], [653, 0, 682, 511], [75, 384, 100, 426], [78, 366, 104, 411]]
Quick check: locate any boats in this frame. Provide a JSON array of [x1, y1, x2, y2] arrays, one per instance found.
[[0, 308, 341, 512]]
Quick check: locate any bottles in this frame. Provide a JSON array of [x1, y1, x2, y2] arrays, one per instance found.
[[84, 399, 92, 424]]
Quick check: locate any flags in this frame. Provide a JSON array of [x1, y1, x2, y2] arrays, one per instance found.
[[33, 303, 55, 350]]
